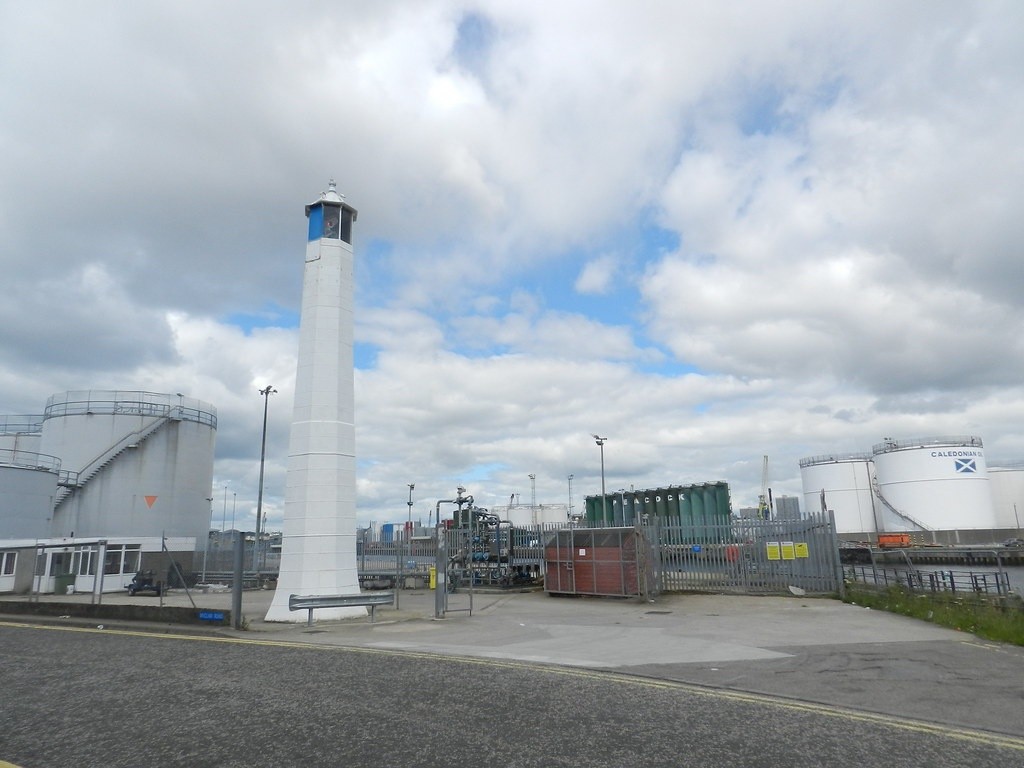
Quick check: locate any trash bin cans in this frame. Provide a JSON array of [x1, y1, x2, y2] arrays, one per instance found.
[[54, 574, 77, 596]]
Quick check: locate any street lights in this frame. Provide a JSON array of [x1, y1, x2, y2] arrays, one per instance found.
[[407, 483, 415, 559], [223, 487, 227, 532], [253, 386, 277, 567], [230, 493, 236, 543], [593, 435, 608, 526]]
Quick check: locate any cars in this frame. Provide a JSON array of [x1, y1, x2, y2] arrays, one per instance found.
[[1004, 538, 1024, 548]]
